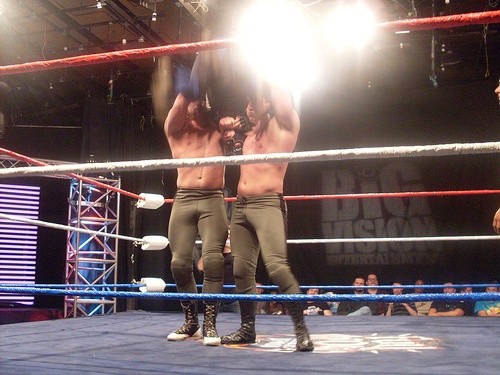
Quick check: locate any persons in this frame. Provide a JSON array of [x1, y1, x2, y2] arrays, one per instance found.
[[196, 189, 500, 317], [164, 51, 234, 346], [492, 78, 500, 235], [221, 73, 315, 352]]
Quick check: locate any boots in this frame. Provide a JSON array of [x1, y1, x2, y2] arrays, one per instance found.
[[167, 299, 201, 341], [220, 301, 257, 344], [285, 301, 314, 351], [202, 300, 222, 346]]
